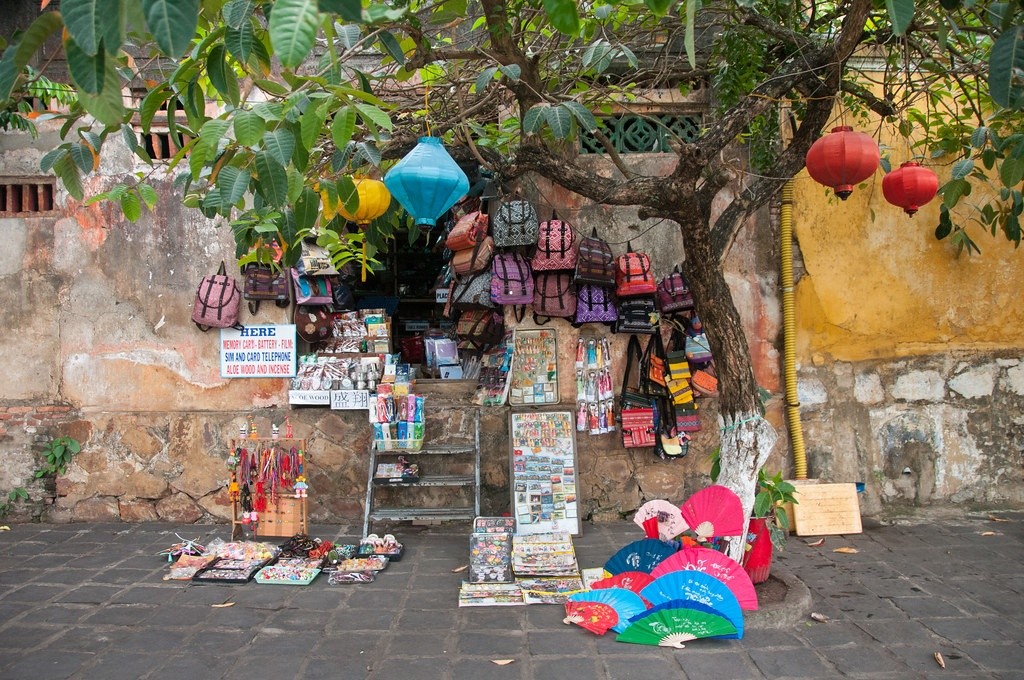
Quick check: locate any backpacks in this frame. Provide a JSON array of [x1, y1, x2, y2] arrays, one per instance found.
[[189, 241, 351, 343], [447, 195, 657, 334]]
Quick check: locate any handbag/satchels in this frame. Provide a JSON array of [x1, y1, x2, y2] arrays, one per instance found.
[[618, 265, 719, 460]]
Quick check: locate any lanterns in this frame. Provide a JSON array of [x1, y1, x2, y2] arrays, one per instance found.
[[330, 168, 393, 230], [384, 132, 470, 233], [806, 124, 881, 203], [882, 159, 939, 220]]
